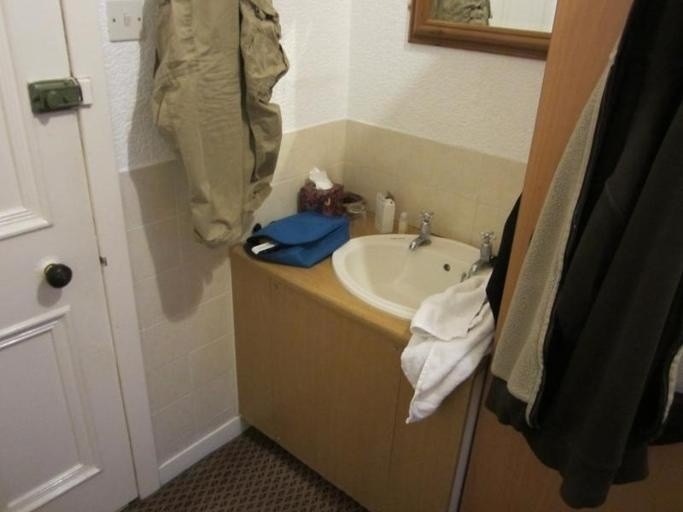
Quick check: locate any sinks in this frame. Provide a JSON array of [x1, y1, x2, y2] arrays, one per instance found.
[[332, 232, 480, 322]]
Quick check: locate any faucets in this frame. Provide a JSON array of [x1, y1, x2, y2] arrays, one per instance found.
[[468, 231, 498, 275], [409, 208, 434, 250]]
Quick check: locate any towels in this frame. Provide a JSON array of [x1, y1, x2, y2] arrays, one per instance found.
[[405, 275, 497, 426]]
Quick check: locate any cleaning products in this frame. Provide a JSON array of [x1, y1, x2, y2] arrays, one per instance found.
[[375, 191, 395, 234]]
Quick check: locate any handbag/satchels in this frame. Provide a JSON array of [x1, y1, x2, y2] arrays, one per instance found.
[[243, 209, 354, 269]]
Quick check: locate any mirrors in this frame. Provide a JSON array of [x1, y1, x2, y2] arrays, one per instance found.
[[404, 1, 556, 64]]
